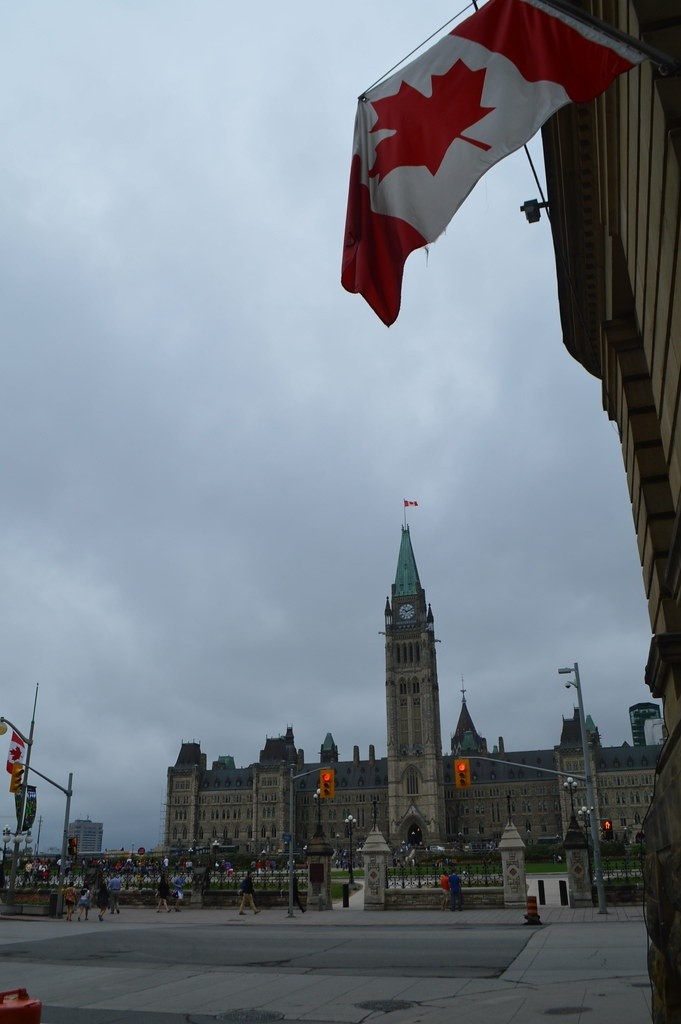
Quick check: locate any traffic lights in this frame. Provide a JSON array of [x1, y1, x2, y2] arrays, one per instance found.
[[68, 836, 77, 855], [9, 762, 25, 793], [454, 758, 471, 790], [603, 820, 614, 839], [320, 769, 335, 799]]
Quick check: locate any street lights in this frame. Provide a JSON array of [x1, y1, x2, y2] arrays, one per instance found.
[[1, 824, 33, 905], [557, 663, 607, 915], [577, 805, 592, 884], [345, 814, 356, 883], [563, 776, 579, 815], [312, 788, 328, 837]]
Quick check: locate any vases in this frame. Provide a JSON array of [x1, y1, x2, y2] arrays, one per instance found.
[[22, 904, 51, 915]]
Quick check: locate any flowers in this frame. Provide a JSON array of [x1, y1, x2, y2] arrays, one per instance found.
[[15, 893, 48, 904]]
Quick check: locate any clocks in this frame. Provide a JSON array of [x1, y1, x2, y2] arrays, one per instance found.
[[400, 604, 415, 620]]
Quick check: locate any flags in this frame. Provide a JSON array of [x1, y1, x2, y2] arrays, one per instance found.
[[341, 0, 647, 327], [7, 732, 26, 774], [405, 500, 418, 506]]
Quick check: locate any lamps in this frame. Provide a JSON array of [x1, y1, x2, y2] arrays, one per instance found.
[[519, 198, 549, 224]]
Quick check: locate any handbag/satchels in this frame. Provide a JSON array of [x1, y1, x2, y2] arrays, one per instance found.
[[171, 891, 178, 899], [155, 891, 160, 899], [238, 888, 244, 896]]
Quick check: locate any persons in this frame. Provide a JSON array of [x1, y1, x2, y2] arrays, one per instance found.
[[115, 855, 169, 882], [174, 871, 185, 912], [56, 857, 71, 875], [77, 885, 90, 921], [436, 857, 452, 867], [288, 874, 307, 913], [90, 858, 106, 870], [155, 875, 176, 913], [238, 870, 260, 915], [220, 859, 234, 876], [439, 870, 464, 911], [23, 857, 51, 882], [108, 873, 120, 914], [95, 878, 108, 921], [552, 853, 562, 863], [65, 882, 78, 920], [251, 858, 275, 872], [174, 857, 187, 872], [336, 858, 362, 870], [392, 857, 400, 866]]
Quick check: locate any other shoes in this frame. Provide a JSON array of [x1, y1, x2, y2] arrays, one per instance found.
[[116, 909, 120, 914], [156, 911, 162, 913], [69, 919, 71, 921], [175, 909, 181, 912], [254, 911, 260, 914], [110, 911, 114, 914], [302, 909, 307, 913], [99, 914, 103, 921], [66, 916, 68, 921], [167, 909, 171, 913], [78, 917, 81, 921], [238, 911, 247, 915], [85, 918, 88, 920]]
